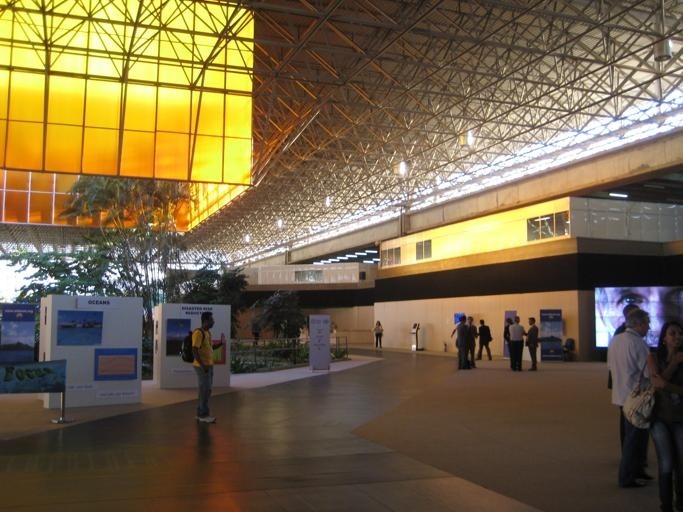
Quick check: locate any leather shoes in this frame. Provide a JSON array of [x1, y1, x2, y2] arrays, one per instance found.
[[618, 471, 655, 489], [528, 366, 539, 372]]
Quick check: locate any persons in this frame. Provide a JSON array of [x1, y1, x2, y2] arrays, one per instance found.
[[503, 318, 518, 369], [475, 319, 493, 360], [449, 315, 468, 369], [463, 316, 479, 368], [605, 308, 655, 488], [251, 323, 261, 346], [188, 311, 216, 424], [507, 315, 527, 371], [607, 305, 648, 469], [524, 317, 538, 370], [643, 320, 682, 511], [372, 321, 383, 347], [595, 287, 682, 347]]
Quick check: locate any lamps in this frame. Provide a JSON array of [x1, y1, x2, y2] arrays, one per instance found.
[[242, 236, 251, 245], [652, 2, 673, 59], [461, 127, 473, 147], [321, 197, 332, 210], [393, 161, 406, 176], [275, 218, 283, 229]]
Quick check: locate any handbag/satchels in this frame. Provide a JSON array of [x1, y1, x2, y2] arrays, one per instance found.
[[623, 386, 655, 429]]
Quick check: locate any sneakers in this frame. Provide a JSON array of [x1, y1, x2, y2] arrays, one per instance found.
[[196, 415, 216, 423]]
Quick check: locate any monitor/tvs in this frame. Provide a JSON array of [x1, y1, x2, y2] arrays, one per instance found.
[[413, 323, 418, 330], [454, 312, 465, 324]]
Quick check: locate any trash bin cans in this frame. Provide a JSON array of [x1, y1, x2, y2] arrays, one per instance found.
[[562, 339, 575, 362]]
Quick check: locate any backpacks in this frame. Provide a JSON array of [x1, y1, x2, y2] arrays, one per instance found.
[[181, 328, 205, 363]]
